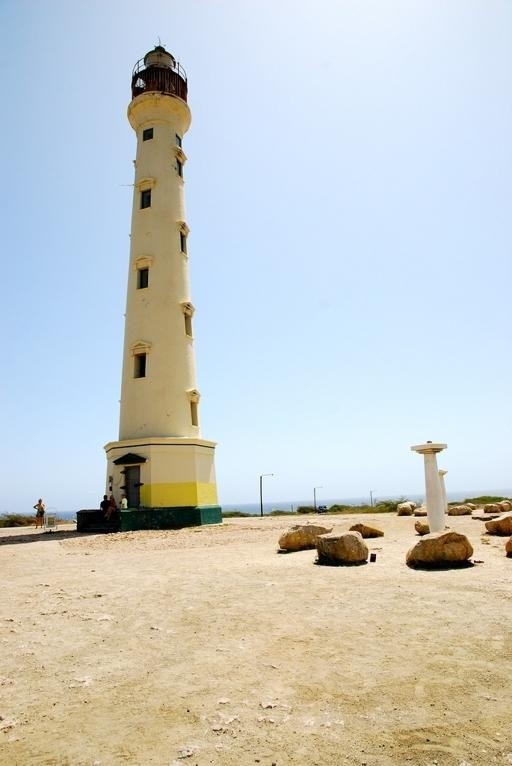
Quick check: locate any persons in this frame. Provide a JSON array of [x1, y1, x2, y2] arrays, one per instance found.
[[119, 491, 130, 507], [108, 495, 118, 532], [34, 499, 45, 528], [99, 494, 109, 533]]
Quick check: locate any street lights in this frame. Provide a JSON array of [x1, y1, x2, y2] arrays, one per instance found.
[[314, 486, 323, 513], [259, 472, 275, 516]]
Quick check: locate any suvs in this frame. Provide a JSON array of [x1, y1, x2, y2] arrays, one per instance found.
[[316, 505, 328, 514]]
